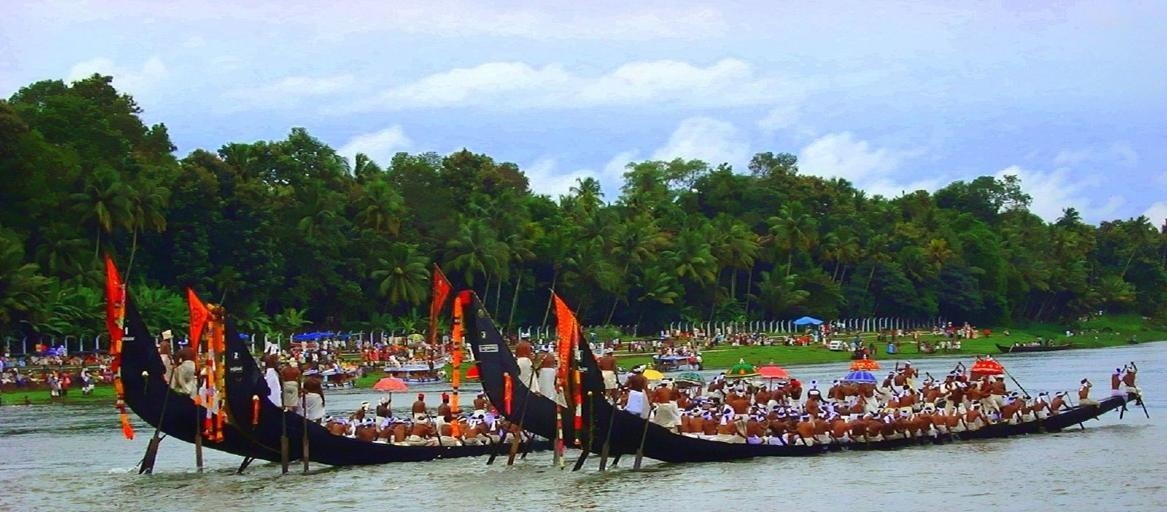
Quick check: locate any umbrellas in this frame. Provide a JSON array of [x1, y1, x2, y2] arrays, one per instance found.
[[791, 316, 824, 330]]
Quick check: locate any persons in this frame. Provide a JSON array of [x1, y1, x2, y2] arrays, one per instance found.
[[1031, 391, 1057, 420], [1011, 392, 1025, 424], [1020, 394, 1037, 423], [1014, 334, 1057, 348], [1109, 364, 1127, 411], [1077, 378, 1100, 422], [1121, 361, 1143, 406], [1050, 391, 1072, 416]]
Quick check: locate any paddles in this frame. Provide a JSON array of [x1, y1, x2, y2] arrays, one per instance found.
[[1066, 393, 1098, 430], [280, 392, 289, 475], [1120, 364, 1149, 419], [634, 410, 651, 469], [599, 403, 621, 471], [196, 369, 202, 473], [573, 451, 589, 471], [137, 367, 175, 474], [486, 422, 512, 465], [508, 371, 534, 465], [236, 457, 255, 474], [303, 394, 309, 472]]
